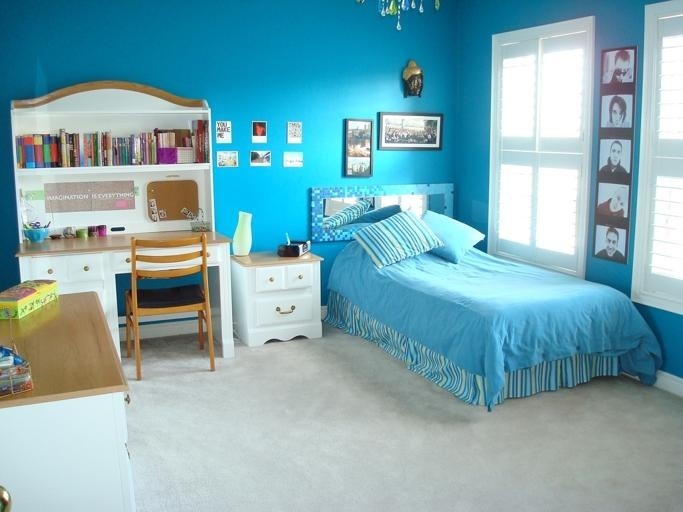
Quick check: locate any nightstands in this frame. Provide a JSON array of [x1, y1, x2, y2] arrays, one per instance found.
[[231, 252, 325, 347]]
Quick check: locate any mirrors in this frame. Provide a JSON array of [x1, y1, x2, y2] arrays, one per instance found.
[[310, 183, 454, 242]]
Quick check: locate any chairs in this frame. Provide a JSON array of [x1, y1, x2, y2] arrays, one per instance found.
[[124, 233, 216, 381]]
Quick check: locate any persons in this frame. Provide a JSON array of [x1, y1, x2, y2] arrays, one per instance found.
[[598, 187, 627, 219], [595, 228, 625, 263], [607, 50, 632, 82], [385, 125, 436, 144], [598, 141, 629, 182], [604, 95, 631, 128]]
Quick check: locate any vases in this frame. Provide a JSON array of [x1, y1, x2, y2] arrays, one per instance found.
[[232, 211, 253, 256]]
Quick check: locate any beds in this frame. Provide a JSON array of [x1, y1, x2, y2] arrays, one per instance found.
[[321, 239, 663, 412]]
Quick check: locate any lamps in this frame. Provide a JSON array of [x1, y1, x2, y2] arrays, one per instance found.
[[359, 0, 440, 31], [403, 60, 423, 97]]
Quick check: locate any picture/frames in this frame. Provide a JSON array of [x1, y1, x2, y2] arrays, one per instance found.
[[344, 118, 373, 177], [592, 45, 637, 265], [379, 112, 442, 150]]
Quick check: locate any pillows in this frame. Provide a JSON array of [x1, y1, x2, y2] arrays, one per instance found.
[[420, 210, 486, 264], [351, 205, 402, 223], [354, 211, 445, 269], [323, 198, 371, 229]]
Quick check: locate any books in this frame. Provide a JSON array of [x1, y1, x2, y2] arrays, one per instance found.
[[15, 119, 210, 168]]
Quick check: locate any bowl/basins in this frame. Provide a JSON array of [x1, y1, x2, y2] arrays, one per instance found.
[[24, 228, 49, 243]]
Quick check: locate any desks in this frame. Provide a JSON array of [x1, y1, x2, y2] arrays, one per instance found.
[[11, 80, 234, 362], [0, 291, 136, 512]]
